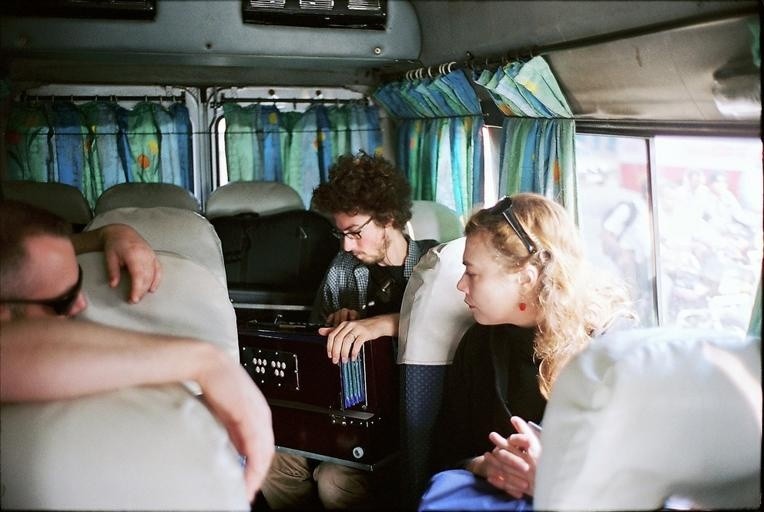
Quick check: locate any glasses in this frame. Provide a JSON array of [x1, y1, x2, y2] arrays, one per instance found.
[[1, 264, 82, 316], [333, 217, 373, 239], [491, 195, 535, 253]]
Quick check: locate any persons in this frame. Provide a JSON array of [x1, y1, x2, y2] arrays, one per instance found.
[[0, 193, 281, 506], [250, 147, 441, 511], [414, 191, 643, 510]]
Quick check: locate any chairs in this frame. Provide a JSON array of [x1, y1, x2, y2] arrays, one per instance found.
[[0, 178, 762, 512]]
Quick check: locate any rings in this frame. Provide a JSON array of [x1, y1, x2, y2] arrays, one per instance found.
[[348, 332, 356, 340]]
